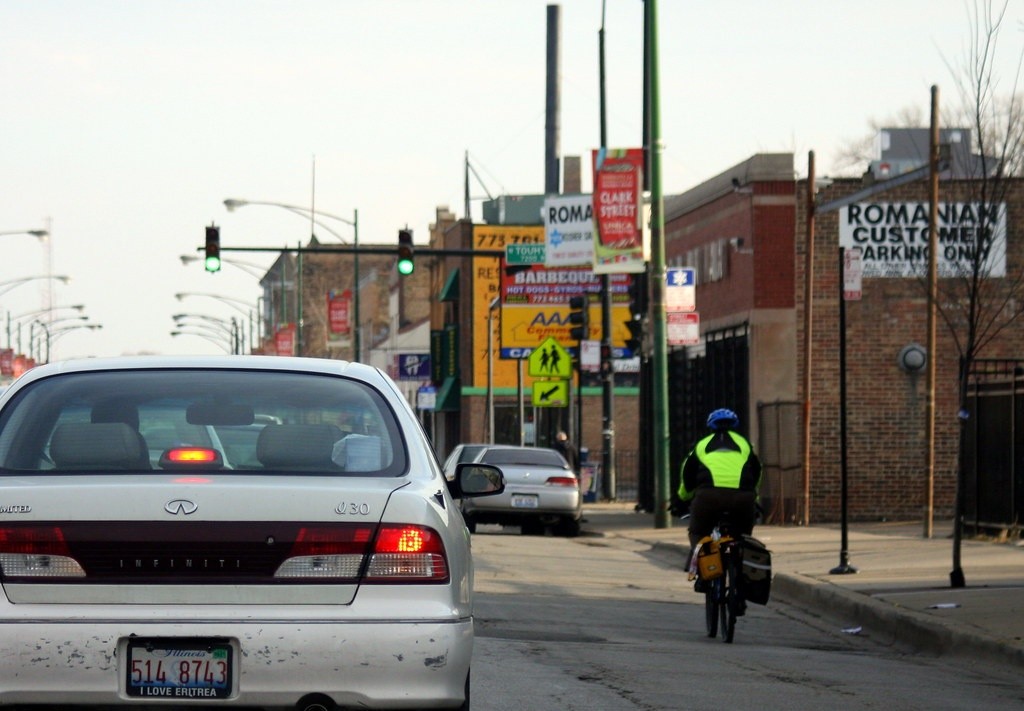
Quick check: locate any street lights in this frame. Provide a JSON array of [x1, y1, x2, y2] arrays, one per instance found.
[[175, 293, 261, 349], [484, 297, 499, 444], [37, 325, 103, 364], [181, 256, 273, 336], [171, 314, 245, 355], [0, 230, 50, 244], [7, 305, 84, 348], [0, 276, 72, 296], [30, 317, 88, 358], [224, 199, 361, 362]]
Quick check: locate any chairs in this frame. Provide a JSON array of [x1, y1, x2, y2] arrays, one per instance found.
[[51, 422, 349, 473]]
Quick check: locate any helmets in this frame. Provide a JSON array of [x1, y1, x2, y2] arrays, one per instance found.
[[707, 409, 739, 427]]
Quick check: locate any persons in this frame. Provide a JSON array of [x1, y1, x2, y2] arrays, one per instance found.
[[548, 431, 578, 482], [666, 409, 762, 617]]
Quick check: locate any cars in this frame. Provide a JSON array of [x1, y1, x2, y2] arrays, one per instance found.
[[212, 414, 282, 470], [45, 407, 233, 474], [0, 354, 506, 711], [442, 443, 513, 507], [461, 447, 582, 536]]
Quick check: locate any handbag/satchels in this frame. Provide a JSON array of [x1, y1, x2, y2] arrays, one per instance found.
[[688, 537, 723, 582], [738, 534, 771, 606]]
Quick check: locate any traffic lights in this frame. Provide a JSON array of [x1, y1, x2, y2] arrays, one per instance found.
[[601, 345, 613, 379], [205, 226, 221, 272], [397, 229, 415, 275], [569, 295, 590, 340]]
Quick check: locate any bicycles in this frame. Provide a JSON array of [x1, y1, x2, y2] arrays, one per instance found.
[[667, 496, 766, 644]]
